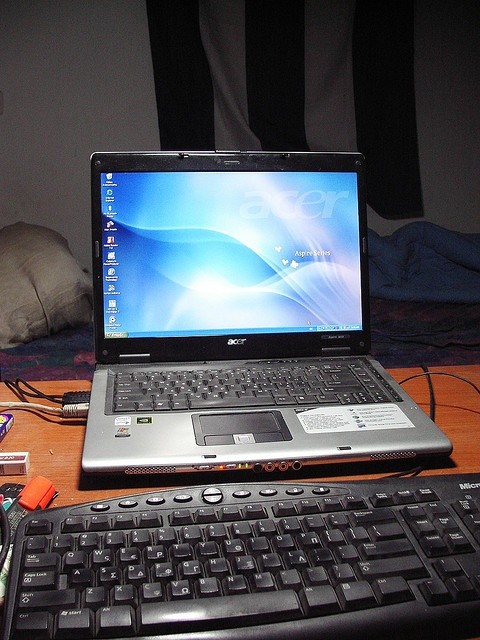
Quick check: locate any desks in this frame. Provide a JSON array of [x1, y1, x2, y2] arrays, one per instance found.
[[0, 364, 480, 639]]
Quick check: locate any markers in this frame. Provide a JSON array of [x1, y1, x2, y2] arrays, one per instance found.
[[1, 475, 57, 545]]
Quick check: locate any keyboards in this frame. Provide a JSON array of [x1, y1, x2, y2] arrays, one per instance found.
[[2, 469, 480, 634]]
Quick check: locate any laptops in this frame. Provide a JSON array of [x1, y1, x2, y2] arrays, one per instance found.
[[80, 149, 455, 475]]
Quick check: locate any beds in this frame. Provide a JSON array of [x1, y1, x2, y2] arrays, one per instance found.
[[0, 221, 478, 380]]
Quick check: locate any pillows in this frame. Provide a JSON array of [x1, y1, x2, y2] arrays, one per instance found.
[[0, 221, 92, 347]]
